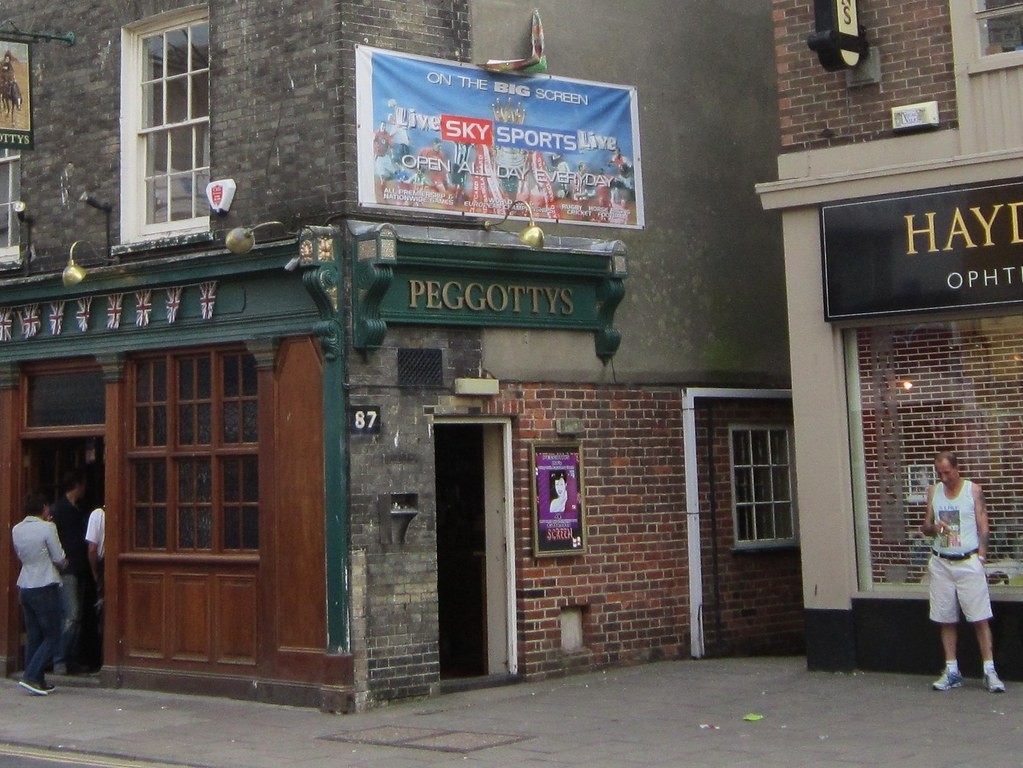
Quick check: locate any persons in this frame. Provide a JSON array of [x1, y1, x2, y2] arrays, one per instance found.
[[50, 473, 90, 676], [921, 450, 1006, 693], [550, 473, 569, 513], [84, 504, 105, 675], [11, 493, 69, 696]]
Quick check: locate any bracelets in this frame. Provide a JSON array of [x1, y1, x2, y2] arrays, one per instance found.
[[978, 555, 987, 562]]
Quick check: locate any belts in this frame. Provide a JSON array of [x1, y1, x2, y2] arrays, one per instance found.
[[932, 547, 978, 561]]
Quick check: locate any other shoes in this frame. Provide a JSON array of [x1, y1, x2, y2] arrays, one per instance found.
[[19, 678, 48, 696], [53, 663, 68, 675], [43, 682, 56, 692]]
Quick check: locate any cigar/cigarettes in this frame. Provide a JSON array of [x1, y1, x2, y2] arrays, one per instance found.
[[940, 526, 944, 533]]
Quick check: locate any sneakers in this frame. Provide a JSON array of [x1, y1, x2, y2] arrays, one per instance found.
[[933, 667, 963, 690], [983, 670, 1004, 692]]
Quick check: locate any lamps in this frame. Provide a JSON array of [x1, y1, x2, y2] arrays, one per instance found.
[[63, 240, 120, 287], [226, 221, 299, 254], [485, 201, 545, 251]]
